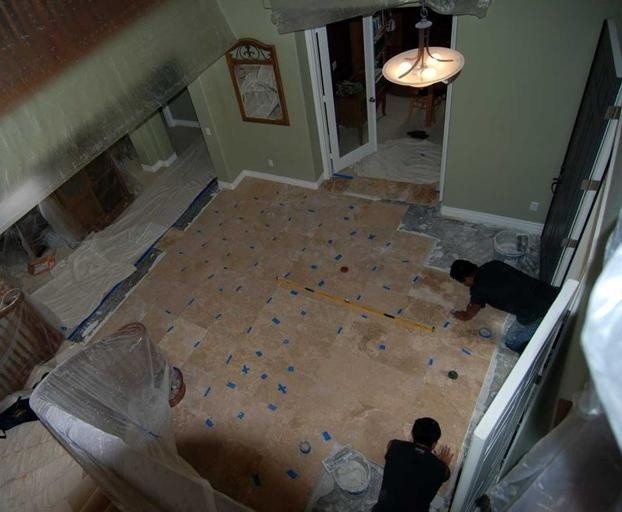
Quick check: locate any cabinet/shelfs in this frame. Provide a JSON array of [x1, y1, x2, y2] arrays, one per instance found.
[[53, 152, 137, 235], [349, 11, 397, 83]]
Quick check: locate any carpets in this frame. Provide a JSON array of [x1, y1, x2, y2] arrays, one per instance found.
[[353, 134, 442, 186]]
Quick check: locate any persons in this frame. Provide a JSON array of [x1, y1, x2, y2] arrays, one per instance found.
[[372, 417, 454, 512], [448, 258, 580, 374]]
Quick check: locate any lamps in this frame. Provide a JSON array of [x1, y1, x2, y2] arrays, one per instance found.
[[381, 1, 467, 90]]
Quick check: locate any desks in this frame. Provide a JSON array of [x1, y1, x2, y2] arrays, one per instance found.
[[334, 67, 387, 146]]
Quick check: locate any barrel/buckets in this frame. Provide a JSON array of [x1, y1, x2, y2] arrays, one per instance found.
[[494, 229, 535, 270], [332, 448, 373, 500]]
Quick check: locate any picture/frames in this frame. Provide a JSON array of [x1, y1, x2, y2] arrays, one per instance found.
[[222, 38, 290, 129]]
